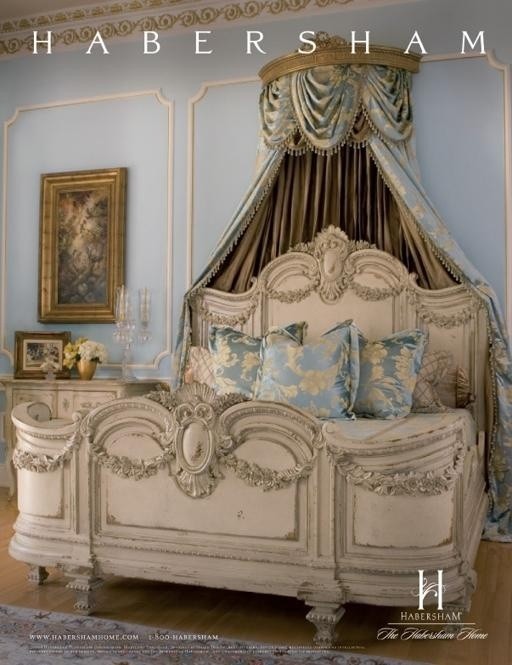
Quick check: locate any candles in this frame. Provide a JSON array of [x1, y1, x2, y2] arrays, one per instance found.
[[109, 281, 151, 326]]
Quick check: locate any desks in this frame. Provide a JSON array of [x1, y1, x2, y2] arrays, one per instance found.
[[0, 376, 163, 501]]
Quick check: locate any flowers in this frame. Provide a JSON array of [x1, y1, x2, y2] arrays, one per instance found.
[[61, 339, 105, 366]]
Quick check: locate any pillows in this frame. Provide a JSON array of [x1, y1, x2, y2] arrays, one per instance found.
[[353, 329, 426, 421], [413, 349, 451, 415], [181, 347, 208, 390], [208, 319, 307, 400], [251, 319, 359, 421]]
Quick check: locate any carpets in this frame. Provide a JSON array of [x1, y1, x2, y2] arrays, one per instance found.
[[0, 603, 409, 665]]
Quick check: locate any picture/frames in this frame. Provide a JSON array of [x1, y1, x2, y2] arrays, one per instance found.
[[12, 331, 73, 380], [37, 168, 126, 325]]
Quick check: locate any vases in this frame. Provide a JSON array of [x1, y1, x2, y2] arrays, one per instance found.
[[77, 360, 98, 381]]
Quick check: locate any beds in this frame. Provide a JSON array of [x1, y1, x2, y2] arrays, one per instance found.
[[10, 226, 490, 647]]
